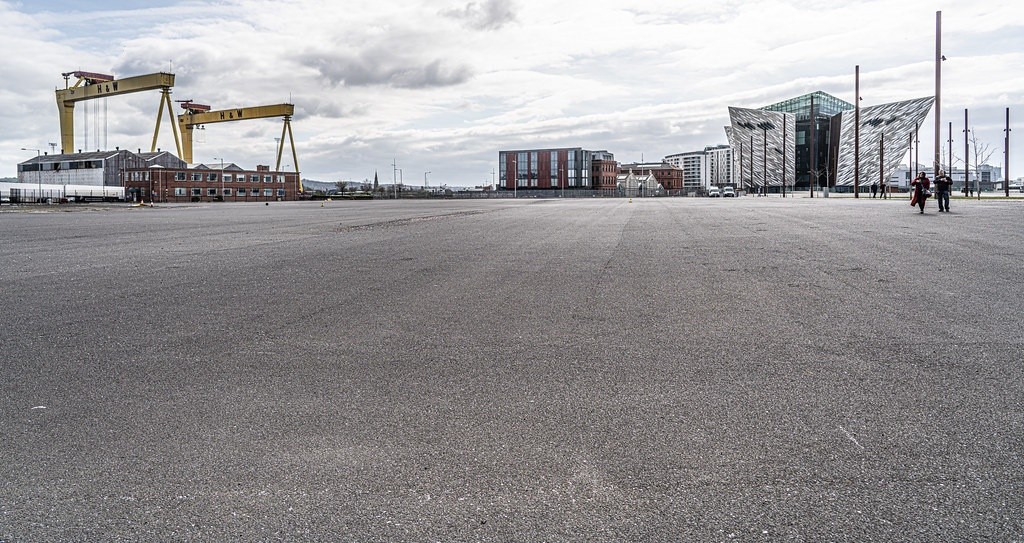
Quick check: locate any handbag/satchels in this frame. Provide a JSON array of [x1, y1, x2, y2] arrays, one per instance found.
[[921, 188, 931, 196]]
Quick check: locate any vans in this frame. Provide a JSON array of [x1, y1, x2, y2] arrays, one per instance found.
[[709, 186, 719, 198], [723, 187, 735, 197]]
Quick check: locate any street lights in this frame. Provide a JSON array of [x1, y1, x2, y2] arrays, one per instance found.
[[21, 147, 42, 206], [425, 171, 432, 187], [214, 157, 225, 201], [395, 168, 402, 184]]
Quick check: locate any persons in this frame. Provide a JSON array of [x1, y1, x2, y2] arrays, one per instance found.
[[910, 172, 930, 214], [871, 182, 887, 199], [933, 169, 953, 212]]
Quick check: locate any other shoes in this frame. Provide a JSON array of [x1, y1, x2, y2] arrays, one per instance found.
[[946, 209, 950, 211], [939, 209, 942, 210], [919, 209, 923, 213]]
